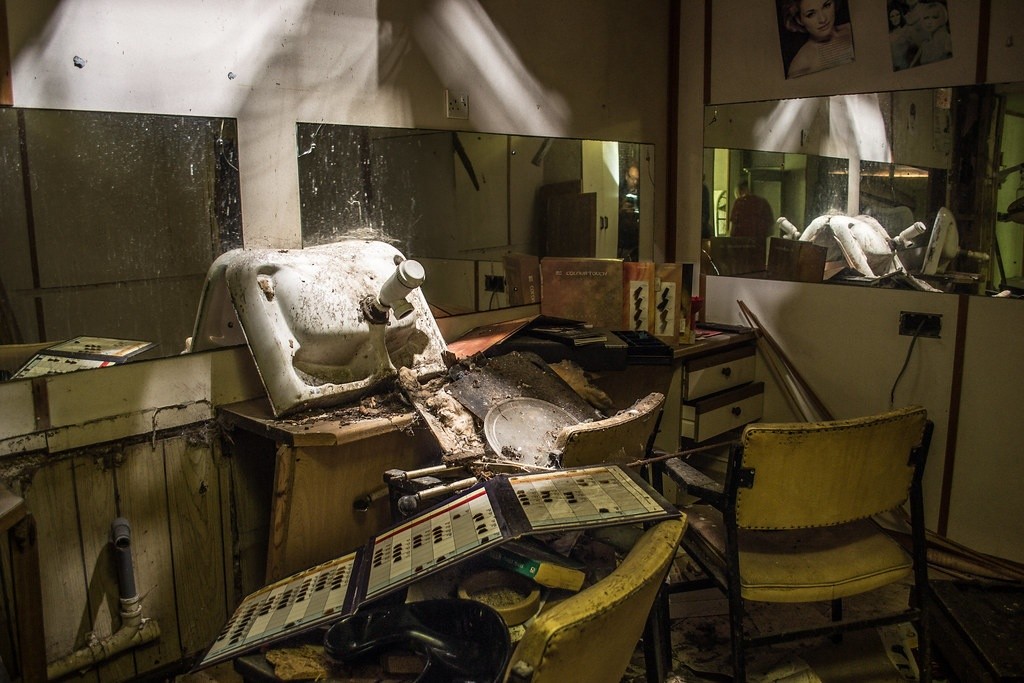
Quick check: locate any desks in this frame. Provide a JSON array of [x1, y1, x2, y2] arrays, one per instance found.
[[413, 239, 546, 315], [215, 320, 764, 607], [923, 578, 1024, 683]]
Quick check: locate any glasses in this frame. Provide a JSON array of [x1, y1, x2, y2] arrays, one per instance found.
[[628, 174, 638, 181]]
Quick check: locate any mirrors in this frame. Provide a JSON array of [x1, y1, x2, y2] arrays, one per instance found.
[[702, 148, 950, 274], [0, 107, 247, 384], [297, 123, 655, 319], [699, 79, 1024, 301]]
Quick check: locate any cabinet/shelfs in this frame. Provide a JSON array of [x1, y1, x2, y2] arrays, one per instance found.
[[544, 137, 619, 260], [652, 339, 763, 506], [0, 277, 215, 363], [0, 107, 214, 290], [371, 131, 545, 256]]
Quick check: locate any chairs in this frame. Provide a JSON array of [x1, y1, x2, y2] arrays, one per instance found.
[[651, 404, 934, 683], [234, 509, 686, 683], [383, 390, 666, 683]]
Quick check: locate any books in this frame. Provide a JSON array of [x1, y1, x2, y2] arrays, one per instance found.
[[483, 541, 588, 594]]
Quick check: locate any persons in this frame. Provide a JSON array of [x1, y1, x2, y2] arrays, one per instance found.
[[620, 165, 640, 212], [785, 1, 856, 77], [727, 180, 775, 238], [889, 3, 955, 70]]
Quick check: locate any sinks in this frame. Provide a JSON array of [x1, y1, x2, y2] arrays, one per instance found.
[[226, 239, 451, 418]]
